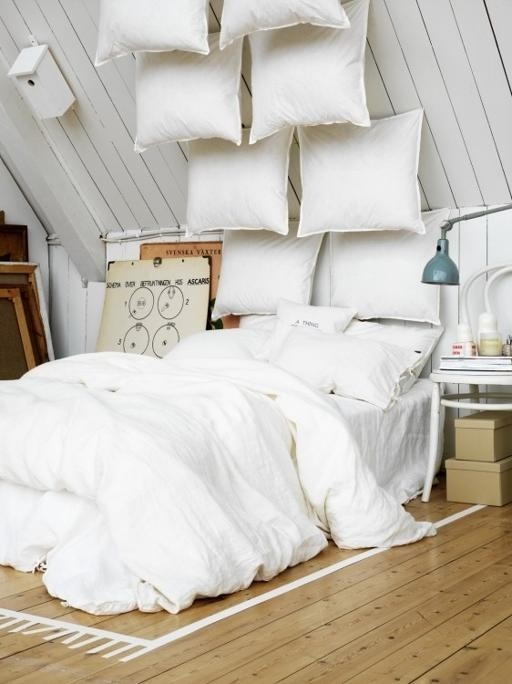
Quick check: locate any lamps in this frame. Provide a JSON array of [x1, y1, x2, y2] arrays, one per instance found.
[[421, 204, 512, 286]]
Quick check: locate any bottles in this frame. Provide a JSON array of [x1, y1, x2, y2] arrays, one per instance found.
[[477, 312, 502, 355], [504, 338, 512, 355], [452, 323, 475, 355]]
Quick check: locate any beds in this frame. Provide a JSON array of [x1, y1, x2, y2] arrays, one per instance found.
[[1, 352, 446, 616]]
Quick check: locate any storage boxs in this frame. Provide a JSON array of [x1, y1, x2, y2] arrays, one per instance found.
[[445, 411, 512, 506]]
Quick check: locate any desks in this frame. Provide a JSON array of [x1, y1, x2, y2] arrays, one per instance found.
[[420, 370, 512, 504]]
